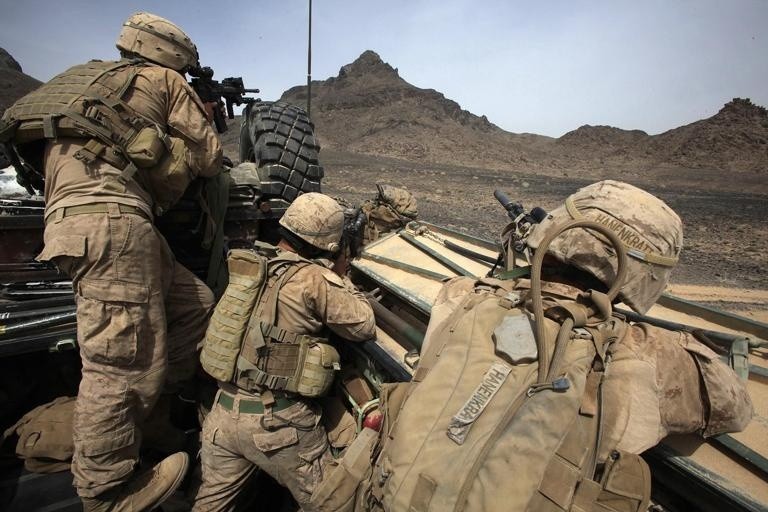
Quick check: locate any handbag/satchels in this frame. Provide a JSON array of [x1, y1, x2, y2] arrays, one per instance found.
[[296, 343, 339, 397], [593, 449, 650, 512], [127, 126, 197, 217]]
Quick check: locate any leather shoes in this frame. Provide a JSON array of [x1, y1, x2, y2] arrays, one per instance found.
[[45, 202, 148, 223], [217, 391, 303, 414]]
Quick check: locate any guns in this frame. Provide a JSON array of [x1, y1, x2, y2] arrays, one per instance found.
[[485, 190, 547, 277], [188, 67, 261, 133], [333, 205, 368, 260]]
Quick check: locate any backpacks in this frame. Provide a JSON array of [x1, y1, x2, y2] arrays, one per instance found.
[[1, 393, 78, 474], [370, 278, 626, 512], [364, 184, 417, 229]]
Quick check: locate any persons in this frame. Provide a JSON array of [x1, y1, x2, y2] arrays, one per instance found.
[[302, 178, 757, 511], [192, 189, 378, 512], [0, 10, 226, 511]]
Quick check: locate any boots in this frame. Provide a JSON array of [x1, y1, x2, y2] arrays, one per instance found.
[[79, 452, 189, 512]]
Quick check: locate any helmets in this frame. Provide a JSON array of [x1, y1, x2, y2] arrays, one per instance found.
[[115, 11, 199, 71], [278, 192, 346, 252], [525, 179, 685, 317]]
